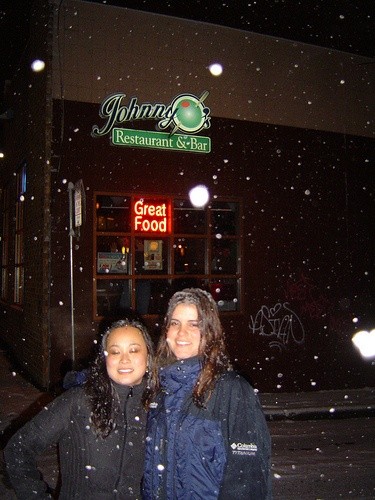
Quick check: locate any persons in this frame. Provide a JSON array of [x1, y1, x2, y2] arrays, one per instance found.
[[64, 289, 273, 500], [3, 320, 155, 500]]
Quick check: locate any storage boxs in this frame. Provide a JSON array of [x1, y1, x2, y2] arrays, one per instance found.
[[208, 283, 237, 303]]
